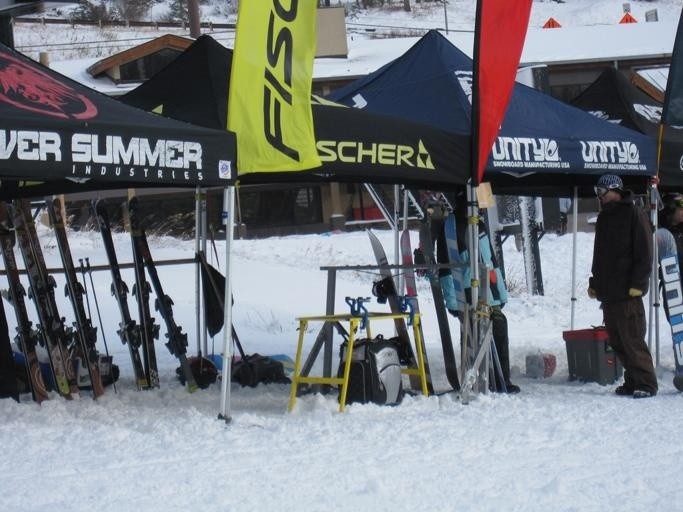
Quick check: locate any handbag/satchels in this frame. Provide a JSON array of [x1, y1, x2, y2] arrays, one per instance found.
[[230, 353, 283, 386], [337, 334, 402, 407]]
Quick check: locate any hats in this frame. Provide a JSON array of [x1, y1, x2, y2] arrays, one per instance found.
[[596, 174, 622, 191]]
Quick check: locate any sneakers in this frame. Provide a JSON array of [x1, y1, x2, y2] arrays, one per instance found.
[[489, 385, 519, 393], [633, 390, 650, 398], [615, 386, 633, 395]]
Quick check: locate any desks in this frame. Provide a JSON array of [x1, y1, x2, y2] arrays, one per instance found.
[[289, 311, 429, 410]]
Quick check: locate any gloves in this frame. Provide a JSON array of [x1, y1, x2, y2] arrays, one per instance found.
[[490, 305, 506, 327], [628, 288, 642, 296], [587, 288, 596, 298]]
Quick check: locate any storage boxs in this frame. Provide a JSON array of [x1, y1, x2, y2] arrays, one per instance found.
[[563, 326, 623, 385]]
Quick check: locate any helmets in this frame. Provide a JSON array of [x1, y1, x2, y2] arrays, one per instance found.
[[176, 357, 216, 390]]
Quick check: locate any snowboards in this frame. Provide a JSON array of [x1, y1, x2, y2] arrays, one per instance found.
[[653, 229, 682, 392]]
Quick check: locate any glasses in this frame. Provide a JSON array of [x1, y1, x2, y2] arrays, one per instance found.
[[597, 183, 622, 198]]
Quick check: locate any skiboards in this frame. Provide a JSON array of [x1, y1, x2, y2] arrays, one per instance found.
[[0, 196, 197, 406], [1, 299, 17, 398], [1, 198, 47, 404], [127, 198, 162, 391], [91, 198, 151, 391], [12, 198, 72, 397], [129, 197, 197, 395], [366, 191, 510, 395], [47, 198, 105, 399], [18, 198, 81, 396]]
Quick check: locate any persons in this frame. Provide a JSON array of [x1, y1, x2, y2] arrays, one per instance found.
[[659, 193, 683, 322], [587, 174, 658, 399], [437, 189, 521, 394]]
